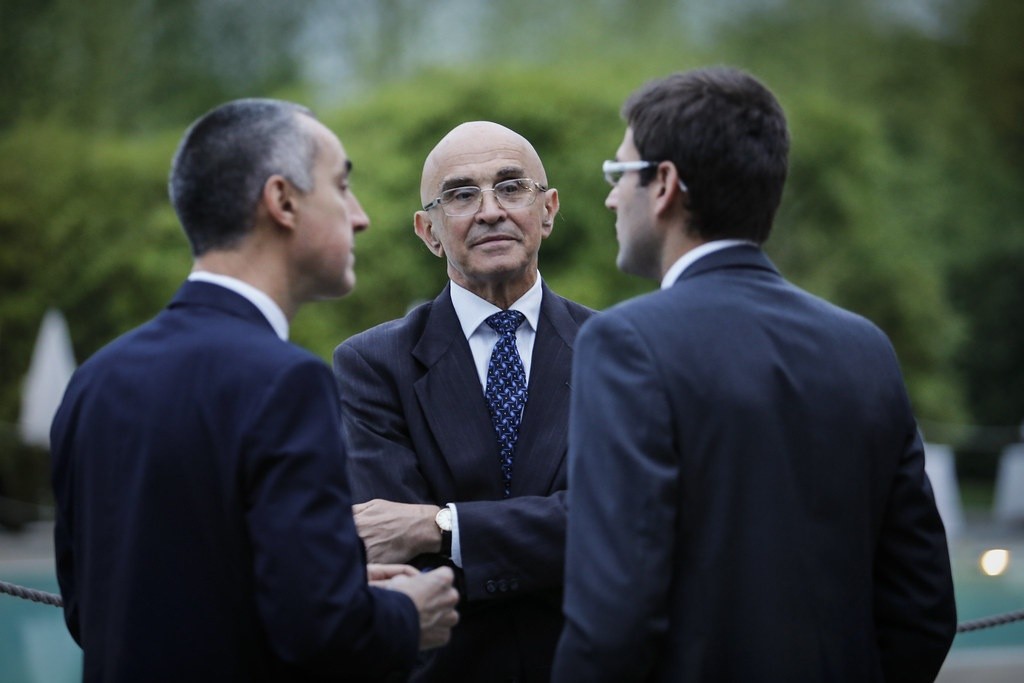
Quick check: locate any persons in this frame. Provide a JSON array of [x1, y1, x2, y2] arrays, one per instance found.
[[47, 98, 459, 683], [336, 120, 597, 683], [555, 67, 958, 683]]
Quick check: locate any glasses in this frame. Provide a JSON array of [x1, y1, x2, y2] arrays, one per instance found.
[[602, 158, 688, 193], [424, 178, 547, 216]]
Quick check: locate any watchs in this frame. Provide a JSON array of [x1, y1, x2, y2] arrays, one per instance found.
[[435, 505, 452, 556]]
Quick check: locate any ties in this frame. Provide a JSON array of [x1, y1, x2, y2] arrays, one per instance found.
[[482, 309, 529, 494]]
